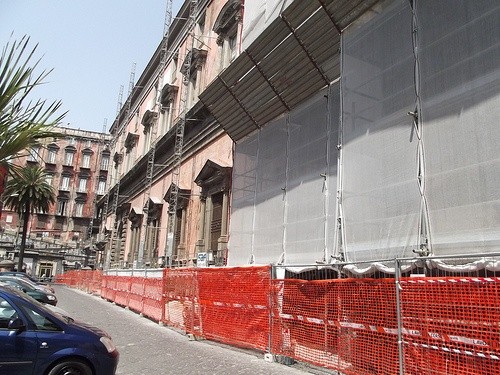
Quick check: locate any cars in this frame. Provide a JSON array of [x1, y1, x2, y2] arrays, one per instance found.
[[0, 271, 48, 292], [0, 276, 58, 308], [0, 282, 119, 375]]
